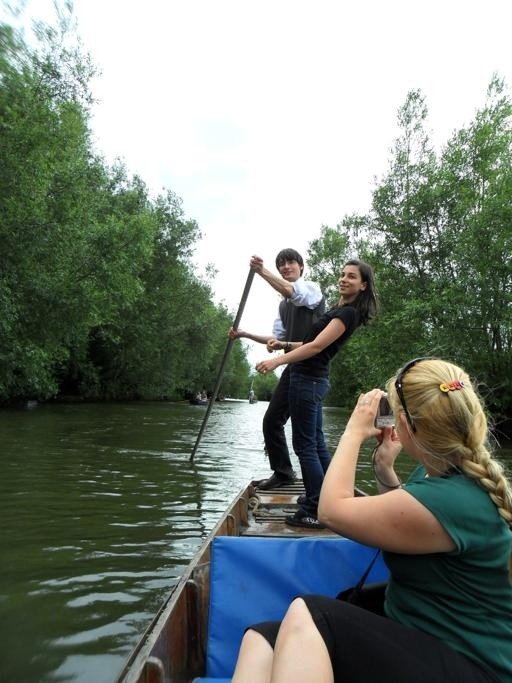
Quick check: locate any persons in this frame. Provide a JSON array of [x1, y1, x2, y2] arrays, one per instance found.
[[194, 390, 207, 402], [229, 354, 511, 683], [225, 247, 334, 489], [255, 259, 378, 531], [247, 387, 254, 403]]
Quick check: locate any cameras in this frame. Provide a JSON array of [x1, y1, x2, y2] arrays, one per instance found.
[[376, 397, 398, 428]]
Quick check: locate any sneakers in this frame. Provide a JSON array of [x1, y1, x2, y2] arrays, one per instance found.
[[285, 510, 319, 528]]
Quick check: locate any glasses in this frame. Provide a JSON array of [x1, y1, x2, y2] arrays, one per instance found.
[[396, 357, 431, 433]]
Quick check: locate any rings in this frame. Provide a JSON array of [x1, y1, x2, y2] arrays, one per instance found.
[[364, 399, 371, 406]]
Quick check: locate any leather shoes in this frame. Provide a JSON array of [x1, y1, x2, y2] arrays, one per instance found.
[[259, 471, 293, 489]]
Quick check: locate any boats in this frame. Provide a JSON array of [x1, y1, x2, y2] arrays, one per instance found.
[[114, 480, 378, 683], [189, 392, 257, 405]]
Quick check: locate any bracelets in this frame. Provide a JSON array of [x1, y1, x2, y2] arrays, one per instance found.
[[286, 342, 293, 351]]
